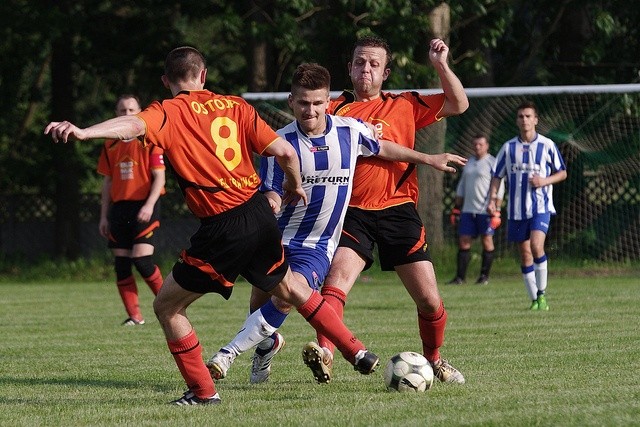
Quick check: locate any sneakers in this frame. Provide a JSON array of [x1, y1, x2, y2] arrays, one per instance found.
[[206, 348, 237, 379], [476, 274, 488, 286], [529, 300, 539, 312], [444, 276, 466, 285], [430, 356, 465, 387], [121, 318, 145, 326], [250, 332, 286, 383], [166, 390, 220, 407], [536, 294, 549, 312], [354, 349, 380, 375], [302, 341, 333, 385]]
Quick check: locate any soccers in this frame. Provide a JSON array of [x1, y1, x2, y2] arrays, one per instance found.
[[385, 351, 434, 394]]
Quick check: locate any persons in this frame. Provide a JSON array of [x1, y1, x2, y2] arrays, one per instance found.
[[96, 95, 167, 327], [445, 134, 506, 287], [300, 36, 470, 386], [208, 63, 467, 383], [44, 46, 381, 407], [487, 102, 567, 312]]
